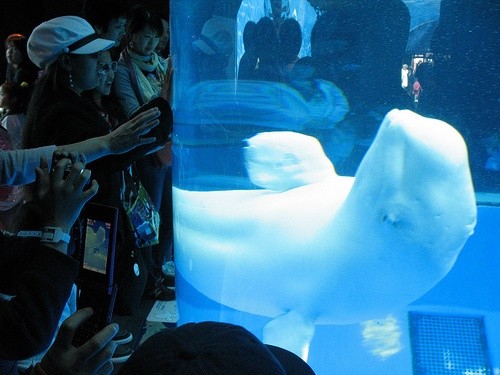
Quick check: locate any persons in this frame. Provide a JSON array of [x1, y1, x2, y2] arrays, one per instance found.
[[192, 11, 235, 79], [0, 4, 315, 375]]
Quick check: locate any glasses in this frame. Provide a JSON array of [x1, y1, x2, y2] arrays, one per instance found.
[[90, 51, 102, 60], [96, 62, 117, 74]]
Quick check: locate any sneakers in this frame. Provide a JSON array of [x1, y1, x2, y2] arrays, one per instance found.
[[111, 345, 134, 363], [144, 281, 175, 301], [112, 328, 133, 345], [156, 271, 175, 286]]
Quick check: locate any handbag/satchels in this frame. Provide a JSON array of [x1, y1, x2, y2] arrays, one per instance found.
[[120, 172, 160, 247]]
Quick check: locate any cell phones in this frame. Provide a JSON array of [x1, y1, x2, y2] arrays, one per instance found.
[[72, 203, 118, 348]]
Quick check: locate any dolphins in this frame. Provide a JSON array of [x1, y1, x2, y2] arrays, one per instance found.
[[168, 104, 479, 363]]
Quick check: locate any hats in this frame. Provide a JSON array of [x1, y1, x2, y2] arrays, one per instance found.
[[118, 321, 316, 375], [27, 16, 115, 69]]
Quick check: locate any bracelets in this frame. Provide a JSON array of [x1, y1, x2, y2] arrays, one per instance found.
[[18, 225, 70, 246]]
